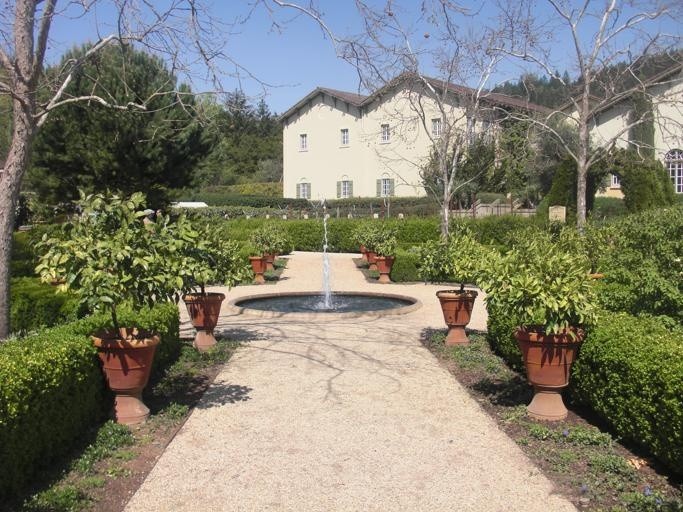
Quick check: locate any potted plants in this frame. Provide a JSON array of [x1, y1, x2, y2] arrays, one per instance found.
[[487, 227, 604, 423], [351, 220, 400, 282], [243, 225, 288, 285], [34, 188, 187, 427], [169, 213, 250, 353], [413, 224, 491, 348]]
